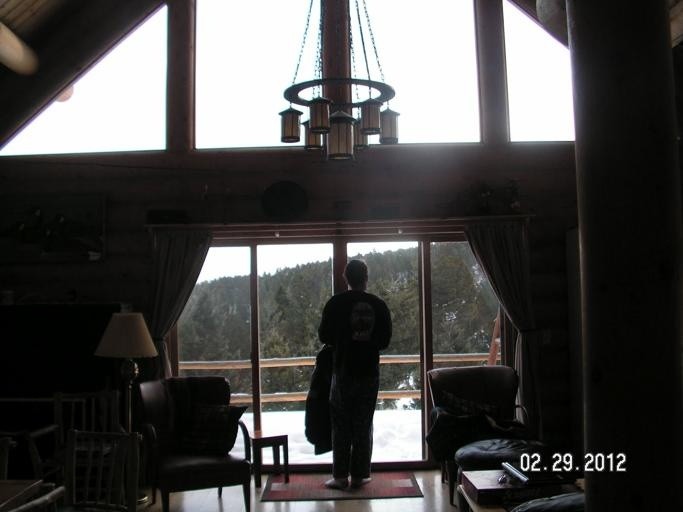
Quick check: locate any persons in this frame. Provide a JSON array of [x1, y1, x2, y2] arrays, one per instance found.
[[318, 257, 392, 492]]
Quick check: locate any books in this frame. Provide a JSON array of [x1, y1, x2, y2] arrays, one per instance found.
[[500, 459, 561, 486]]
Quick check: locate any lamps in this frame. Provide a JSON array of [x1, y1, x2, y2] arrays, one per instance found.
[[279, 0, 398, 163], [93, 312, 162, 505]]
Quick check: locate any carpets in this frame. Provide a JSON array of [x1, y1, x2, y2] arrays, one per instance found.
[[260, 470, 422, 500]]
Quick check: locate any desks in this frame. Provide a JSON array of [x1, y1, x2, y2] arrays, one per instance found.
[[457, 479, 585, 512], [0, 478, 66, 512]]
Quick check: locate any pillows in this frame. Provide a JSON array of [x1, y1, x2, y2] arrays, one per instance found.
[[169, 404, 248, 456]]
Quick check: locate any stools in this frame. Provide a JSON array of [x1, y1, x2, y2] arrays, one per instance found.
[[246, 430, 289, 488], [457, 438, 548, 512]]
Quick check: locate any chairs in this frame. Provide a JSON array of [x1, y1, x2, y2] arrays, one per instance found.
[[426, 366, 530, 507], [25, 389, 126, 505], [63, 430, 142, 512], [135, 374, 251, 512]]
